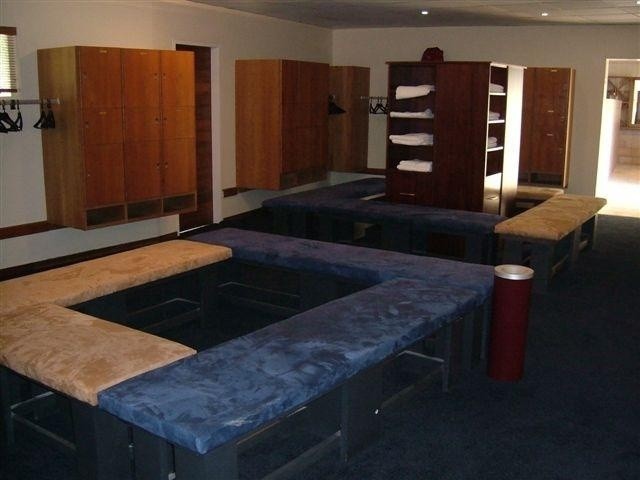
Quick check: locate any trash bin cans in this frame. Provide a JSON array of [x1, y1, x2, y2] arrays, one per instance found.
[[486, 264, 534, 381]]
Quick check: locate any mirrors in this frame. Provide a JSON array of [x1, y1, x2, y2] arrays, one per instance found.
[[625, 77, 640, 130]]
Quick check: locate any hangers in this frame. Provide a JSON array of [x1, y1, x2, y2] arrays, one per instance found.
[[0, 99, 23, 134]]
